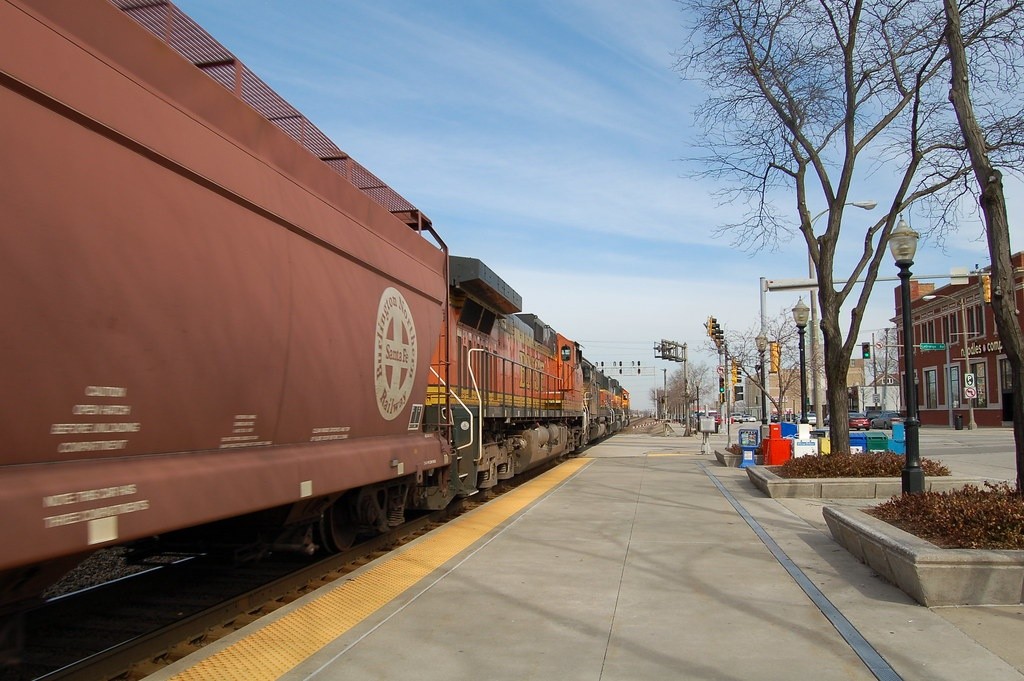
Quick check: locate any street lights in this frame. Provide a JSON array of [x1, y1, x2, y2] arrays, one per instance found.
[[888, 212, 926, 498], [807, 200, 878, 453], [921, 294, 978, 431], [754, 332, 769, 426], [792, 297, 810, 423]]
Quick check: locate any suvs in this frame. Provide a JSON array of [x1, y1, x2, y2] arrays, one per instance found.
[[731, 412, 744, 424]]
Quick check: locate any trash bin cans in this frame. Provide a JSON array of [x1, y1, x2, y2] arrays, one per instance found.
[[849, 432, 866, 454], [955, 414, 964, 430], [863, 431, 888, 453]]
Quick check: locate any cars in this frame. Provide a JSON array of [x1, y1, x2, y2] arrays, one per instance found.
[[866, 409, 884, 420], [742, 415, 757, 422], [846, 413, 870, 431], [770, 410, 817, 425], [870, 413, 920, 429], [823, 414, 829, 426]]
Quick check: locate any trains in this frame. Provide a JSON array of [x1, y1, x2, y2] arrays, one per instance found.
[[0, 0, 632, 627]]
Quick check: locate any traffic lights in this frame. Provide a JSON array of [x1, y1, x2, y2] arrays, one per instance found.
[[709, 316, 725, 348], [719, 377, 724, 393], [732, 362, 742, 384], [861, 341, 872, 359]]
